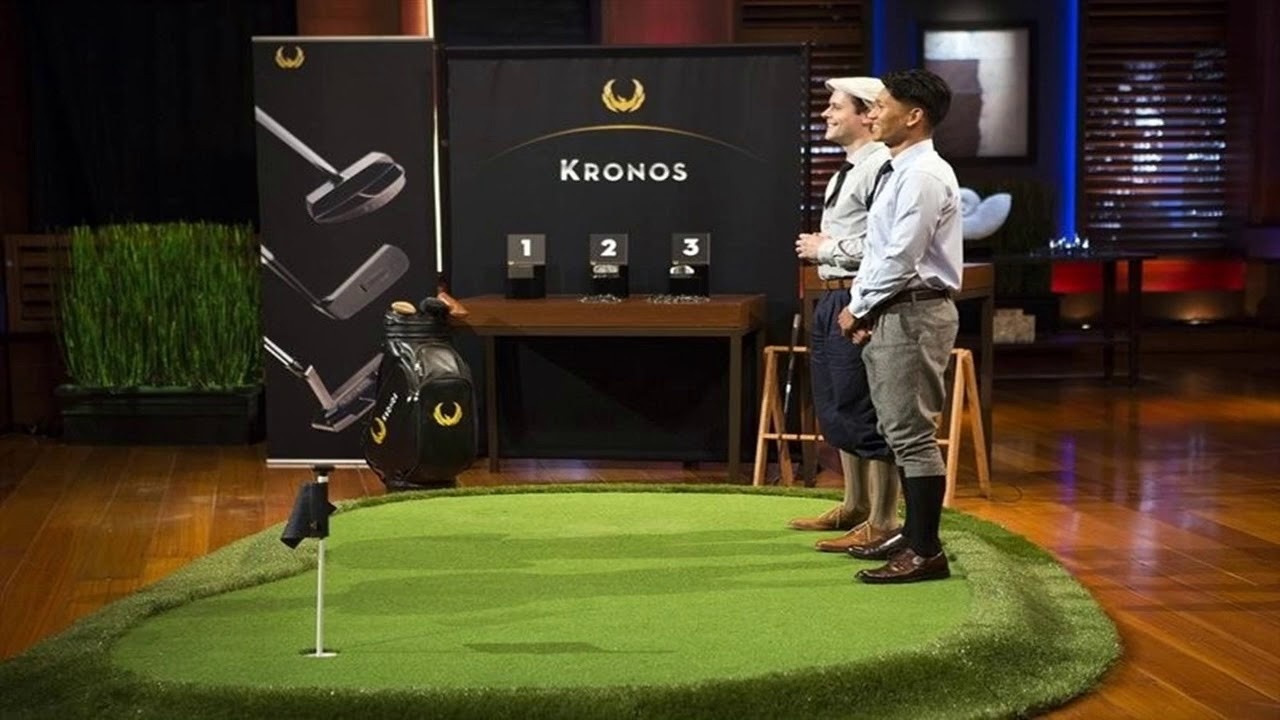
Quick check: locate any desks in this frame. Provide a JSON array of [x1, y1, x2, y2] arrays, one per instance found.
[[448, 293, 769, 482]]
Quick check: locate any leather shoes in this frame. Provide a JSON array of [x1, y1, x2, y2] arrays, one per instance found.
[[787, 504, 870, 531], [854, 546, 950, 584], [848, 526, 911, 560], [815, 522, 904, 551]]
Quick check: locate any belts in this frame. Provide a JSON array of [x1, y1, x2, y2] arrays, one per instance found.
[[818, 276, 859, 291], [879, 285, 953, 306]]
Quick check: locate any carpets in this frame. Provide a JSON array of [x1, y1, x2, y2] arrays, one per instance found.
[[0, 484, 1124, 720]]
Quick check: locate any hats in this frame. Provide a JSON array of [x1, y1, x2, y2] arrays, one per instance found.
[[825, 77, 883, 103]]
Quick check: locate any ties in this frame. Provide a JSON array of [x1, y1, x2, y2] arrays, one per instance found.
[[825, 161, 852, 209], [865, 158, 892, 212]]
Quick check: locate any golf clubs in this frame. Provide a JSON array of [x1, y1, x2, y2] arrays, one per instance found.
[[260, 244, 411, 321], [256, 89, 409, 227], [264, 333, 384, 434]]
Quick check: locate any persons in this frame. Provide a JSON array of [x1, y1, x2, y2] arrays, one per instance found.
[[788, 77, 894, 552], [833, 71, 964, 585]]
